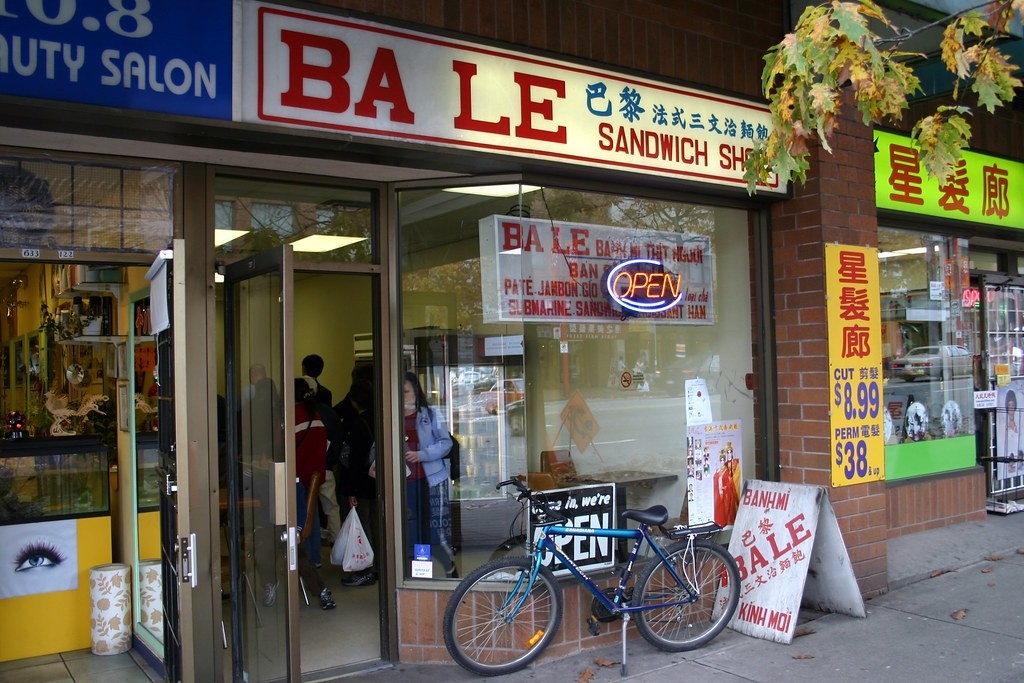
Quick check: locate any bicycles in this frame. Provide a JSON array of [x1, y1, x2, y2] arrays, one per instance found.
[[441, 473, 741, 678]]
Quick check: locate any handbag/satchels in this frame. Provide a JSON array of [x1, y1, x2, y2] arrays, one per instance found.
[[441, 431, 470, 480], [330, 506, 375, 572]]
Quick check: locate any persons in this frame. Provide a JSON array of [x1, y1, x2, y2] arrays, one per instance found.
[[402, 372, 459, 578], [244, 354, 376, 612]]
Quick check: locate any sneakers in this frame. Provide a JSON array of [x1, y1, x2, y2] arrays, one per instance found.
[[320, 590, 336, 609], [341, 573, 374, 586], [264, 577, 279, 607]]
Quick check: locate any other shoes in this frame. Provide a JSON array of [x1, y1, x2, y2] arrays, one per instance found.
[[446, 566, 458, 578]]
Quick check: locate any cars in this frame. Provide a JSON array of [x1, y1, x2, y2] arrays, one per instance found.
[[479, 378, 526, 416], [449, 365, 496, 394], [502, 397, 526, 436], [656, 352, 720, 396], [888, 344, 974, 383]]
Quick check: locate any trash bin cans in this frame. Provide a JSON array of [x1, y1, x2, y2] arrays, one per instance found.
[[89, 564, 131, 656]]
